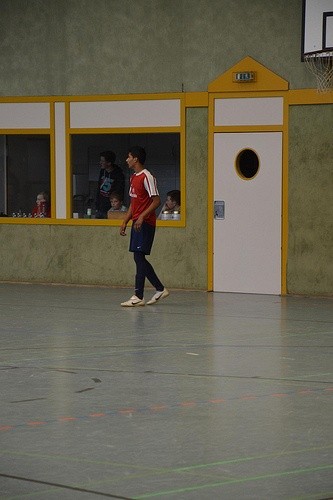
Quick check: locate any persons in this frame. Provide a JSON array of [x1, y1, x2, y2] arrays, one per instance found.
[[106, 192, 127, 218], [31, 191, 50, 218], [118, 146, 169, 306], [157, 190, 182, 219], [92, 150, 125, 218]]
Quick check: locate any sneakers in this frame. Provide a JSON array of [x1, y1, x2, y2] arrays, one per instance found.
[[147, 288, 170, 305], [120, 295, 145, 307]]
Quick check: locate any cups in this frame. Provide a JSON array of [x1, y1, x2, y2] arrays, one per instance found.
[[87, 208, 92, 215], [73, 213, 78, 218]]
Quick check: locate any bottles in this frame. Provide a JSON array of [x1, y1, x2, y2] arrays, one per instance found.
[[172, 211, 181, 220], [12, 213, 44, 217], [161, 211, 172, 220]]
